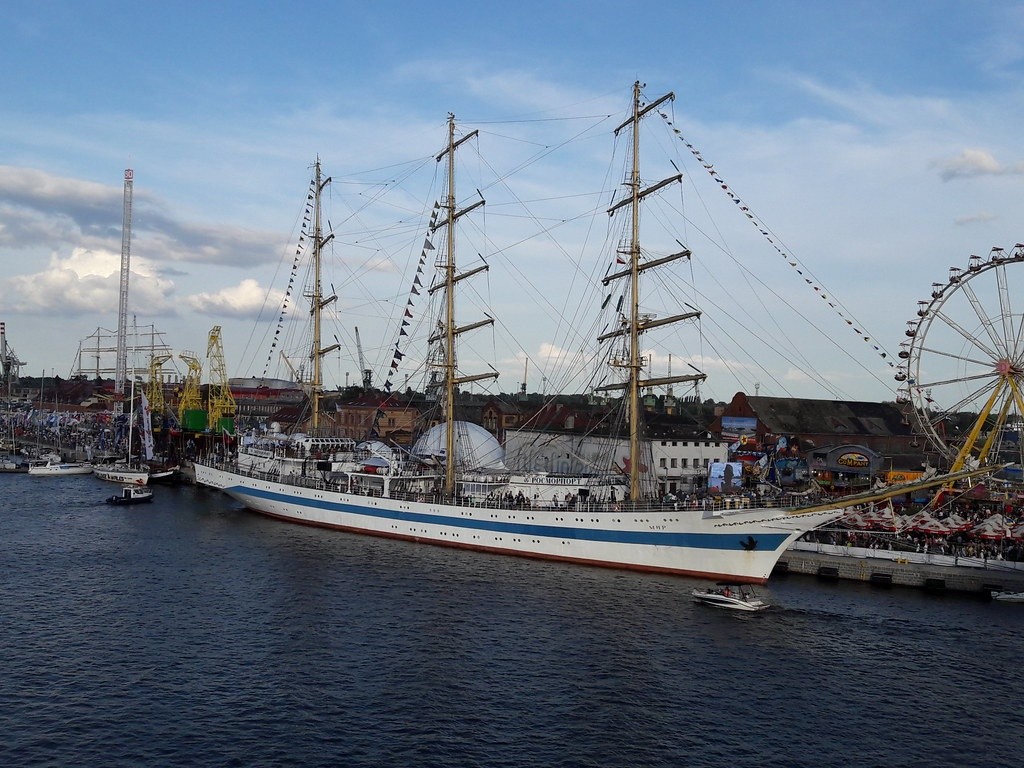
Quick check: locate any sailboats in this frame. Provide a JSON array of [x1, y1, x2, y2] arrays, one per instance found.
[[191, 82, 1016, 586], [0, 327, 185, 485]]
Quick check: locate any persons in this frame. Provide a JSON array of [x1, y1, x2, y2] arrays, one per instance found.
[[166, 427, 830, 520], [706, 585, 735, 599]]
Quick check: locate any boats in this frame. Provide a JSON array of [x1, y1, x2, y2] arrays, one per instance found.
[[690, 581, 771, 613], [105, 484, 154, 505], [990, 590, 1024, 604]]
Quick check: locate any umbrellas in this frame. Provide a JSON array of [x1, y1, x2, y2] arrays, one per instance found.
[[803, 506, 1024, 556]]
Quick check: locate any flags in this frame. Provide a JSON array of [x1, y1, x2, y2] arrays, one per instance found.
[[14, 410, 105, 432], [142, 389, 156, 460]]
